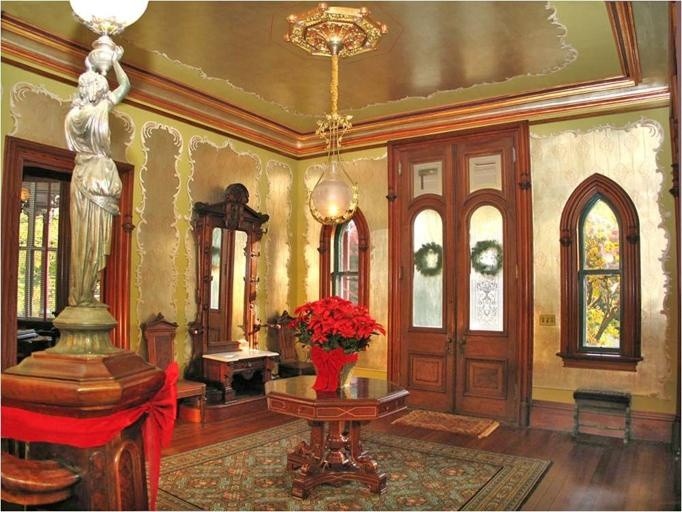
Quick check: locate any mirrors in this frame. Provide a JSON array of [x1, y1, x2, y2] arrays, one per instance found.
[[206, 228, 249, 351]]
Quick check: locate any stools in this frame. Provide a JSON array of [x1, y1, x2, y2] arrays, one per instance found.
[[573, 387, 635, 446]]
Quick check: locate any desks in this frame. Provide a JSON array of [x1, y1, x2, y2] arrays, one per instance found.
[[200, 347, 281, 406], [263, 376, 410, 499]]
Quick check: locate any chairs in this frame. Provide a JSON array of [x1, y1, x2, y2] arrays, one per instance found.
[[140, 312, 208, 427], [270, 310, 317, 378]]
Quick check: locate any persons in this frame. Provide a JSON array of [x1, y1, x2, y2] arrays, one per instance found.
[[63, 45, 133, 309]]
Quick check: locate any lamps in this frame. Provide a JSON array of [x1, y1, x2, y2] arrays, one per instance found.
[[68, 0, 151, 33], [279, 4, 390, 229], [19, 185, 30, 211]]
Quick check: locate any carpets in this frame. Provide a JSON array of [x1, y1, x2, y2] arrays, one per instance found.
[[392, 408, 501, 441], [146, 414, 555, 511]]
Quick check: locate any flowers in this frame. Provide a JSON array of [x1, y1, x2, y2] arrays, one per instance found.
[[286, 299, 390, 352]]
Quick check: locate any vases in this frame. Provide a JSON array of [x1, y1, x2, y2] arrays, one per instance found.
[[308, 348, 356, 389]]
